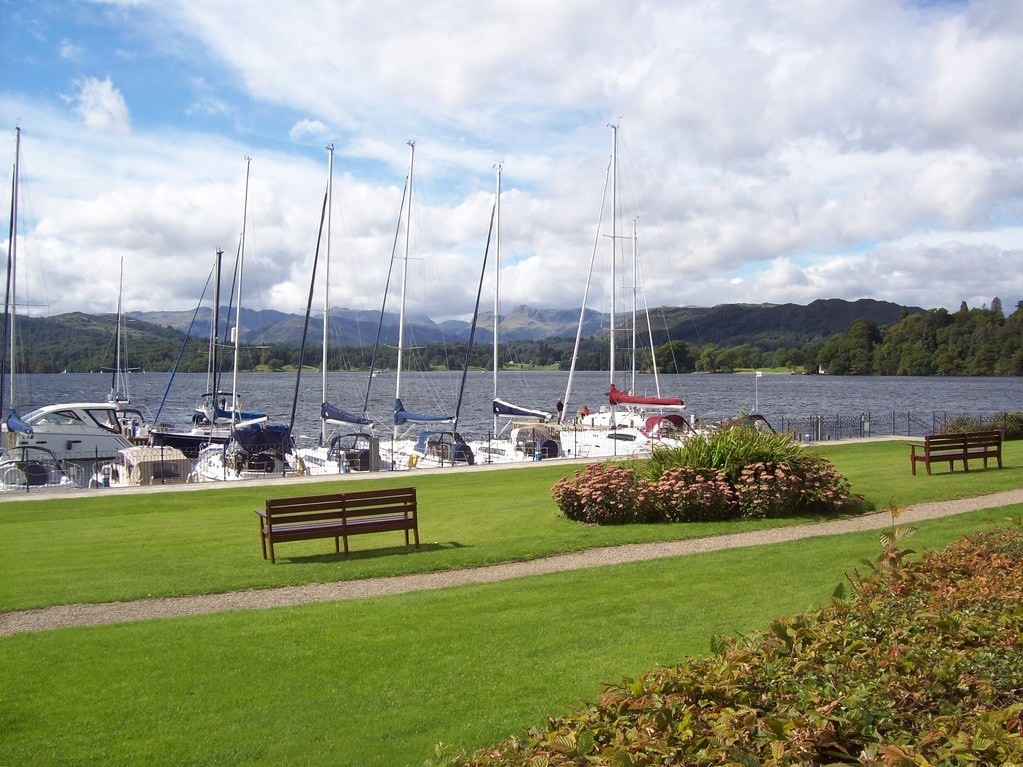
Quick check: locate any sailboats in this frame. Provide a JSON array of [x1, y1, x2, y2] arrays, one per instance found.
[[195, 153, 310, 480], [446, 159, 566, 464], [0, 122, 158, 491], [95, 255, 173, 446], [544, 117, 779, 454], [284, 145, 404, 473], [352, 139, 479, 470], [145, 247, 260, 457]]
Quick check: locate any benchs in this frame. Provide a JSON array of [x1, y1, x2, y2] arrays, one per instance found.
[[907, 430, 1003, 477], [254, 486, 421, 564]]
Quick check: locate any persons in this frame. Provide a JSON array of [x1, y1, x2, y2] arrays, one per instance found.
[[580, 405, 589, 419], [556, 398, 563, 421]]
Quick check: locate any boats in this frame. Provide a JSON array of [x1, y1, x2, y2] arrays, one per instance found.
[[0, 443, 90, 494], [90, 443, 193, 489]]
